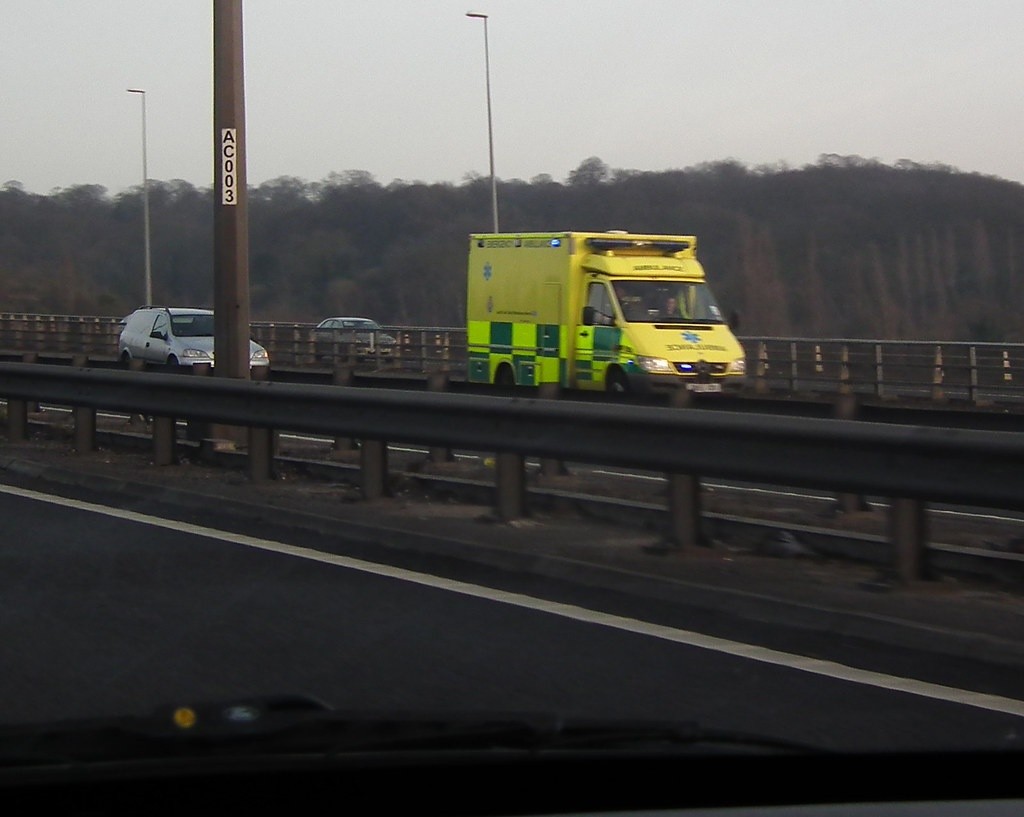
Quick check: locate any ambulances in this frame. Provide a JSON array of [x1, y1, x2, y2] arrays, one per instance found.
[[466, 231, 749, 404]]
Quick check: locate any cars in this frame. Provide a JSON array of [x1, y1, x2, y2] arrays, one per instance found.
[[119, 307, 271, 380], [314, 318, 397, 363]]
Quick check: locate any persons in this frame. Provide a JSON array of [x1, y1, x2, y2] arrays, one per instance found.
[[608, 286, 636, 319], [659, 297, 682, 319]]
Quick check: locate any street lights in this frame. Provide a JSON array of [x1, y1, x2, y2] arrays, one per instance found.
[[466, 11, 500, 234], [127, 88, 152, 306]]
